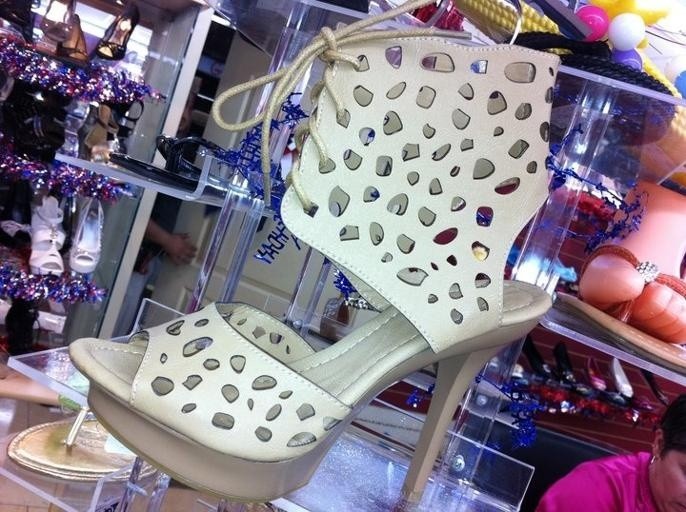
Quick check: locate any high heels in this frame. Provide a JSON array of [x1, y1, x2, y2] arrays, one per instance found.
[[77, 95, 144, 161], [3, 0, 140, 68], [0, 74, 69, 166]]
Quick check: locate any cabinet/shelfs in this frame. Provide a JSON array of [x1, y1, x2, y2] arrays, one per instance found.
[[0, 0, 685, 512]]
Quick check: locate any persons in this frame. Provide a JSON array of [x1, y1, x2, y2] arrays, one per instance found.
[[145, 77, 201, 265], [535, 390, 686, 512]]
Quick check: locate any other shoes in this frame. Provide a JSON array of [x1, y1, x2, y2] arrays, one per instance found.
[[4, 299, 42, 355], [67, 196, 106, 275], [2, 176, 32, 248]]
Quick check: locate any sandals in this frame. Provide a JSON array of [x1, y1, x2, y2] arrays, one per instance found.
[[29, 196, 66, 278], [107, 134, 269, 205], [71, 2, 555, 503]]
